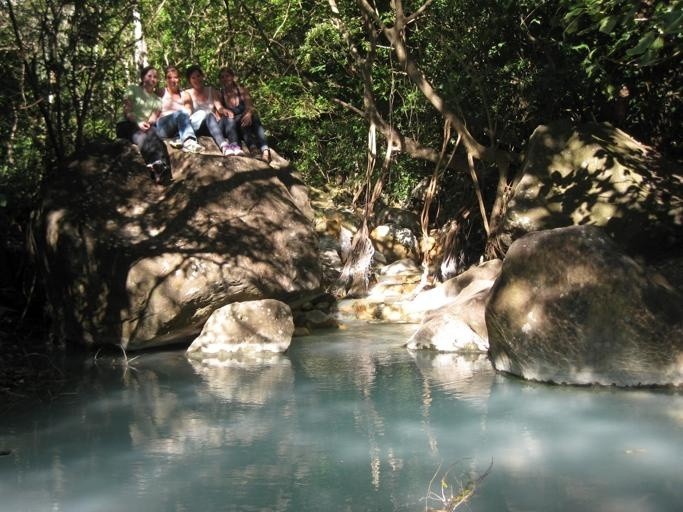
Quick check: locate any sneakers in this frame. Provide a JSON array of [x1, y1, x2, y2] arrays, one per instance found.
[[262, 150, 290, 170], [223, 144, 245, 156], [169, 138, 183, 148], [183, 140, 204, 153], [147, 160, 171, 183]]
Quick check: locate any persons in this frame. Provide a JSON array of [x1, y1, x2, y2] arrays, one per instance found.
[[112, 67, 173, 185], [153, 65, 206, 154], [182, 65, 244, 158], [215, 66, 272, 162]]
